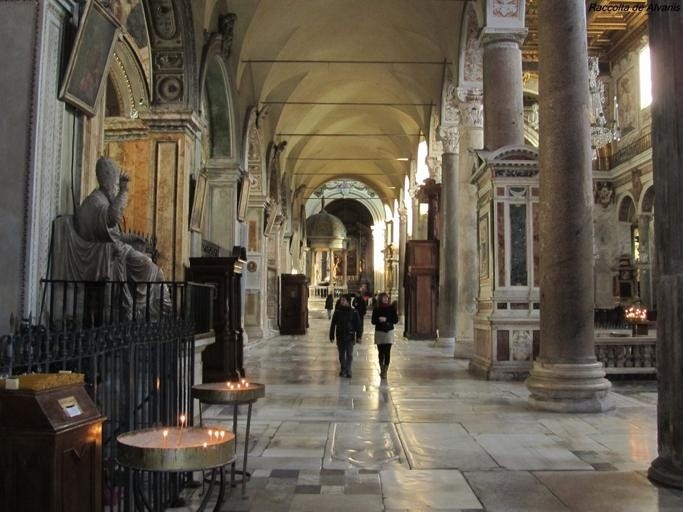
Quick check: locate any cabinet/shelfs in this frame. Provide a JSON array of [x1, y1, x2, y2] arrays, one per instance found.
[[281, 273, 310, 335], [404, 241, 439, 341], [188, 246, 248, 382]]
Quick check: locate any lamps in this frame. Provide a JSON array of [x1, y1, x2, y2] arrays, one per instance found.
[[587, 55, 615, 151]]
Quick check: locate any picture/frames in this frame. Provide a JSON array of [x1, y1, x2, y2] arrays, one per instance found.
[[58, 0, 121, 118], [264, 205, 279, 237], [189, 174, 208, 233], [237, 176, 251, 222]]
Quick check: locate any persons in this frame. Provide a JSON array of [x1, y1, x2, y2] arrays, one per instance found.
[[329, 294, 362, 378], [72, 157, 170, 318], [371, 293, 398, 378], [335, 292, 377, 343], [325, 293, 333, 320]]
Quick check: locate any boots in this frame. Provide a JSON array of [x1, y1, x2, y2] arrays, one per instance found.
[[379, 362, 388, 379]]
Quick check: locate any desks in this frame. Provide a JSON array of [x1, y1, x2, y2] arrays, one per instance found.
[[116, 426, 236, 511], [192, 381, 264, 500]]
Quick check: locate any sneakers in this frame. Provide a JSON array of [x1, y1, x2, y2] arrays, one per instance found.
[[339, 367, 352, 377]]
